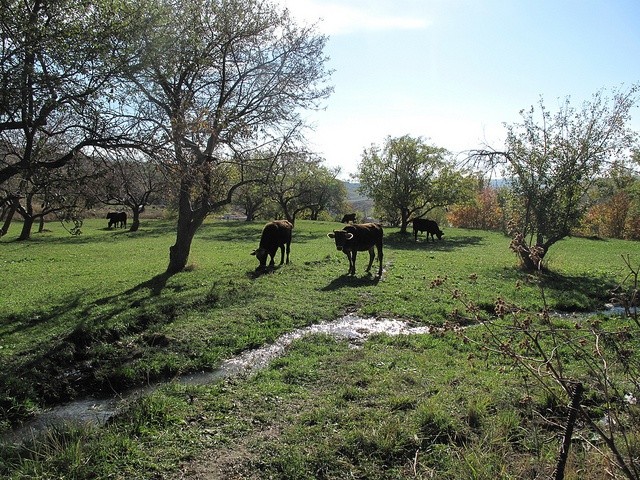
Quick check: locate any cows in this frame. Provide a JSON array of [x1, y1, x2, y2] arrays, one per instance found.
[[341, 213, 356, 224], [108, 212, 127, 229], [250, 220, 293, 267], [327, 223, 383, 277], [106, 212, 120, 228], [413, 218, 444, 242]]
